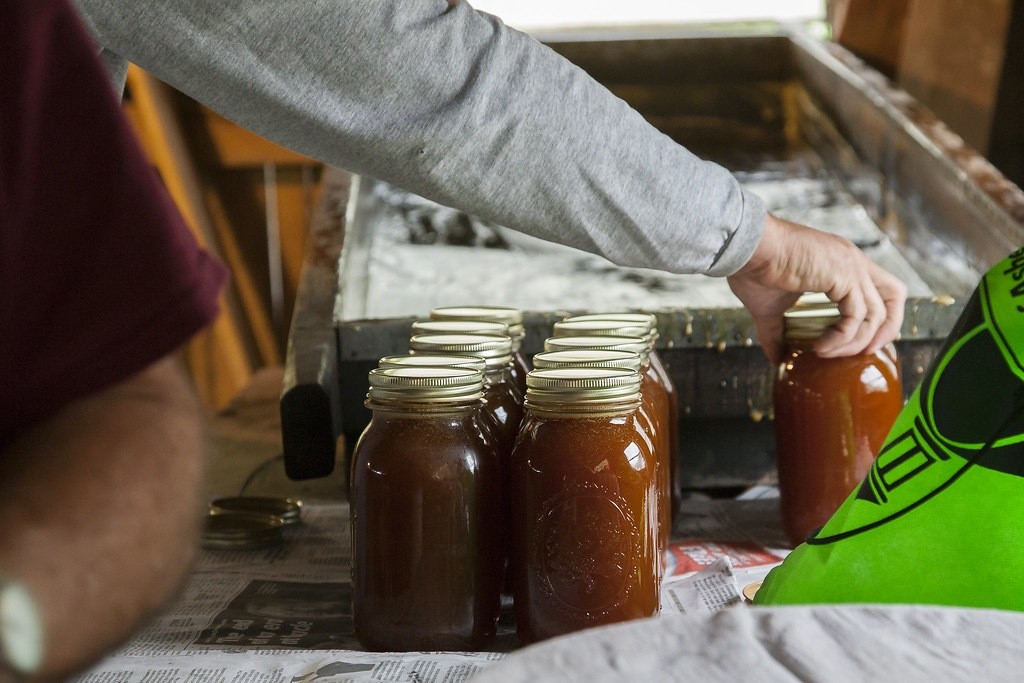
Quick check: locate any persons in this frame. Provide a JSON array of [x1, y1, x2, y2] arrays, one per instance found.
[[0, 1, 229, 683], [753, 239, 1024, 610], [71, 0, 906, 357]]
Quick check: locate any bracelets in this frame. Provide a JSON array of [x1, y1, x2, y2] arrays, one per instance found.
[[0, 587, 45, 675]]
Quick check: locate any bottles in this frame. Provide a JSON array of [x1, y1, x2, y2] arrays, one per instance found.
[[774, 306, 900, 546], [347, 308, 679, 652]]
